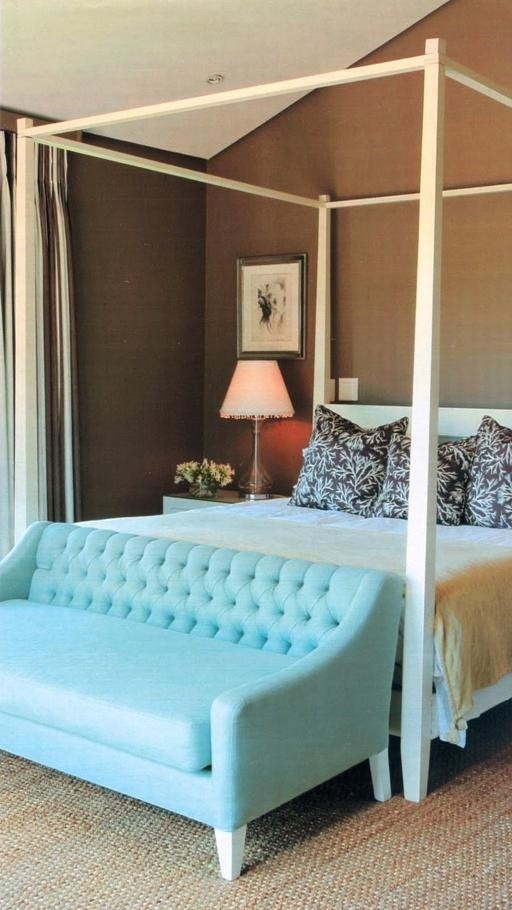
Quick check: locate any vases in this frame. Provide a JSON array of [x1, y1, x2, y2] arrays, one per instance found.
[[174, 458, 234, 502]]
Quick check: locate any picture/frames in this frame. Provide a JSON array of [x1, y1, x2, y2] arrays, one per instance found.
[[236, 251, 305, 363]]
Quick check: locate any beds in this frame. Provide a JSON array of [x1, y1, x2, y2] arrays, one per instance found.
[[73, 407, 512, 804]]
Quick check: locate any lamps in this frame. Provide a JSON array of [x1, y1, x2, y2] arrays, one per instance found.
[[218, 359, 295, 499]]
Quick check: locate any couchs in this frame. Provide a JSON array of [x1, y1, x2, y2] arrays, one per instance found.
[[0, 478, 203, 520], [2, 517, 405, 885]]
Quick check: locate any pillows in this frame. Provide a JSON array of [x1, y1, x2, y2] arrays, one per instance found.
[[288, 405, 512, 530]]
